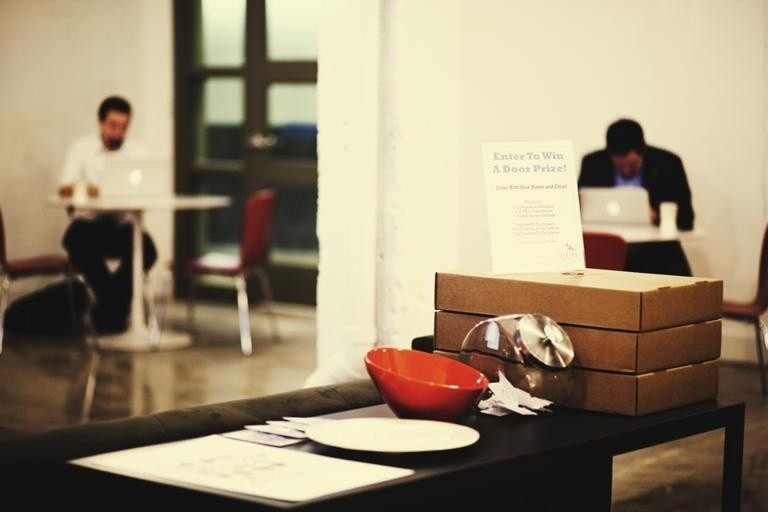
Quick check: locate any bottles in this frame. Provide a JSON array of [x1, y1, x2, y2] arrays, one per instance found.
[[460, 312, 575, 425]]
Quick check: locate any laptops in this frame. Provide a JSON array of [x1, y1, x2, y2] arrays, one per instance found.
[[100, 151, 173, 200], [580, 187, 654, 227]]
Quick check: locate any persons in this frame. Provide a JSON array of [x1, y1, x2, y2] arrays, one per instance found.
[[577, 118, 695, 277], [60, 95, 158, 334]]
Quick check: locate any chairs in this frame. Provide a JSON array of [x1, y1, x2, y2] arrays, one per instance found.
[[183, 189, 278, 355], [582, 229, 628, 271], [0, 211, 73, 348], [719, 221, 767, 408]]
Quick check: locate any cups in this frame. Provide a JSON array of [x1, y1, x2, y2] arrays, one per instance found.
[[658, 201, 678, 237]]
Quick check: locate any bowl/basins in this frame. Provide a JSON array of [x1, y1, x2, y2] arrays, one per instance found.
[[366, 347, 490, 421]]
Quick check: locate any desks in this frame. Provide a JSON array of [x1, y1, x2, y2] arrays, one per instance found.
[[2, 366, 742, 512], [44, 193, 231, 351], [581, 221, 702, 250]]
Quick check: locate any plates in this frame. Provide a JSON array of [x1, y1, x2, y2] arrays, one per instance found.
[[303, 417, 481, 454]]
